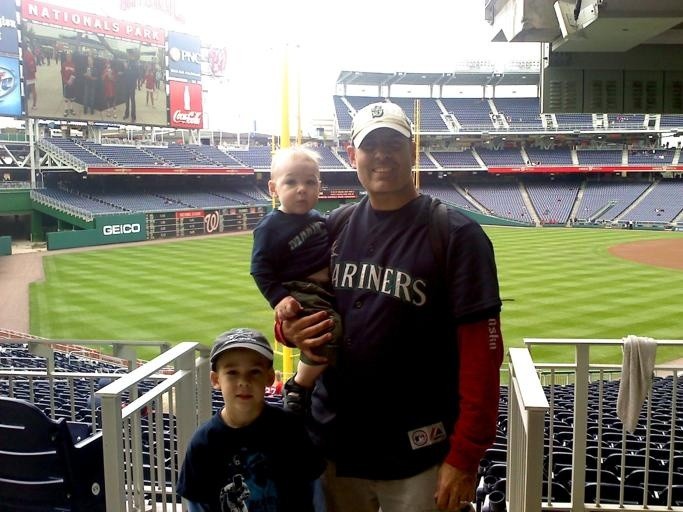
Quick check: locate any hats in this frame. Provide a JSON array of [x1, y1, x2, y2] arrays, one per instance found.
[[209, 327, 273, 362], [351, 99, 412, 148]]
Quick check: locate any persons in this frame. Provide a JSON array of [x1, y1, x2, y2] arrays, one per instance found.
[[268, 370, 283, 396], [250, 146, 343, 426], [176, 328, 328, 512], [275, 102, 503, 511], [22, 36, 166, 122]]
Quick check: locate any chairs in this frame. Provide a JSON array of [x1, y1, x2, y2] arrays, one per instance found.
[[2, 338, 682, 505]]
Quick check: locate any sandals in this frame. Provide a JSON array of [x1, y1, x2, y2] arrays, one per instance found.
[[280, 373, 313, 413]]
